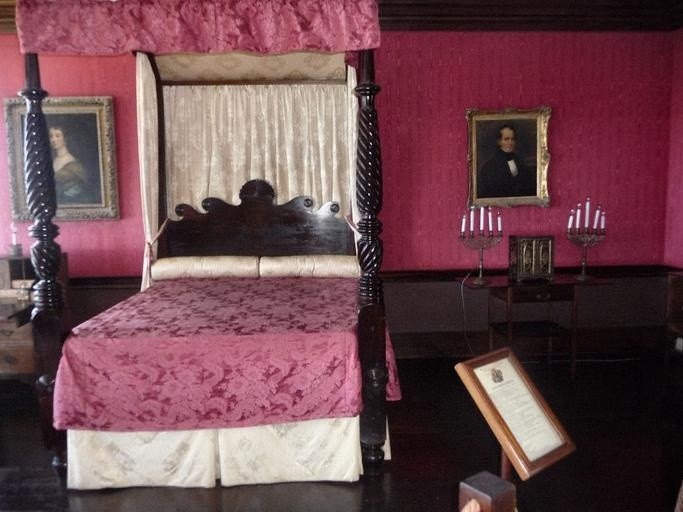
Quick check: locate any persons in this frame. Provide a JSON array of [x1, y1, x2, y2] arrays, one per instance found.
[[482, 126, 527, 194], [49, 126, 89, 201]]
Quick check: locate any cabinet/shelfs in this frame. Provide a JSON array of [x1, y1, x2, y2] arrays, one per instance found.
[[0, 300, 36, 381], [664, 271, 683, 338], [1, 252, 68, 290]]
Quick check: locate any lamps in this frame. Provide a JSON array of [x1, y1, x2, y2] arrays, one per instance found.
[[460, 205, 504, 286], [566, 197, 606, 281]]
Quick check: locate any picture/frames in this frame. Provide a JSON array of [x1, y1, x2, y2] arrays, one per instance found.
[[466, 106, 553, 208], [4, 95, 122, 222], [454, 345, 577, 484]]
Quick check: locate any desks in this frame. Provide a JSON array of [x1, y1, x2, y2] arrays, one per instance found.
[[458, 268, 609, 386]]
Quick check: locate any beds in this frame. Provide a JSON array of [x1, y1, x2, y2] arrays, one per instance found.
[[52, 179, 403, 490]]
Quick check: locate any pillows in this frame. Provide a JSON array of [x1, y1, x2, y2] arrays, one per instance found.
[[259, 253, 362, 280], [151, 253, 260, 281]]
